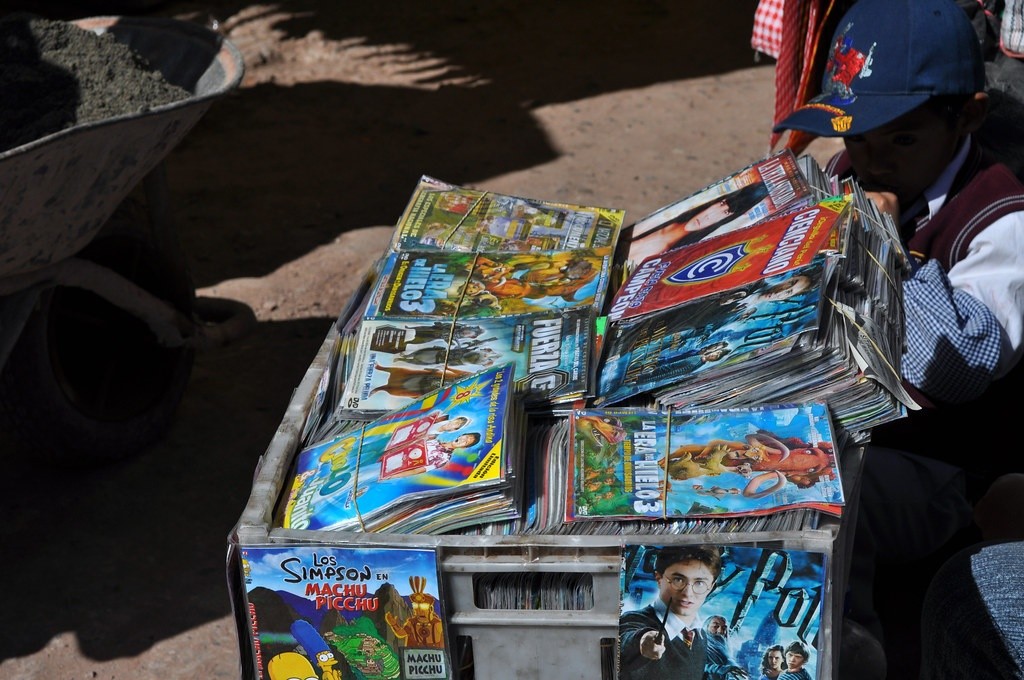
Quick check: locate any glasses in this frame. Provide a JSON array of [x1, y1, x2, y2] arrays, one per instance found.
[[660, 574, 718, 594]]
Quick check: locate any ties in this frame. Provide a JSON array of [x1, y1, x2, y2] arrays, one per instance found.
[[681, 627, 694, 649]]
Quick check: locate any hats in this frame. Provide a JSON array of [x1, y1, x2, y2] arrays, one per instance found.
[[773, 0, 985, 137]]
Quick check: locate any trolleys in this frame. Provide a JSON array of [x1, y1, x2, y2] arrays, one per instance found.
[[0, 13, 245, 482]]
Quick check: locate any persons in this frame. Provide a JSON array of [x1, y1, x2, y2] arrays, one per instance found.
[[386, 432, 482, 478], [628, 181, 774, 264], [758, 641, 814, 680], [620, 544, 721, 680], [650, 266, 822, 331], [769, 0, 1024, 569], [428, 410, 472, 438], [658, 341, 729, 364], [636, 348, 732, 385], [703, 615, 750, 680]]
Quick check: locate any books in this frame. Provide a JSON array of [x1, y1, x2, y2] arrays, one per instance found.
[[276, 147, 922, 611], [617, 544, 828, 680], [238, 546, 454, 680]]
